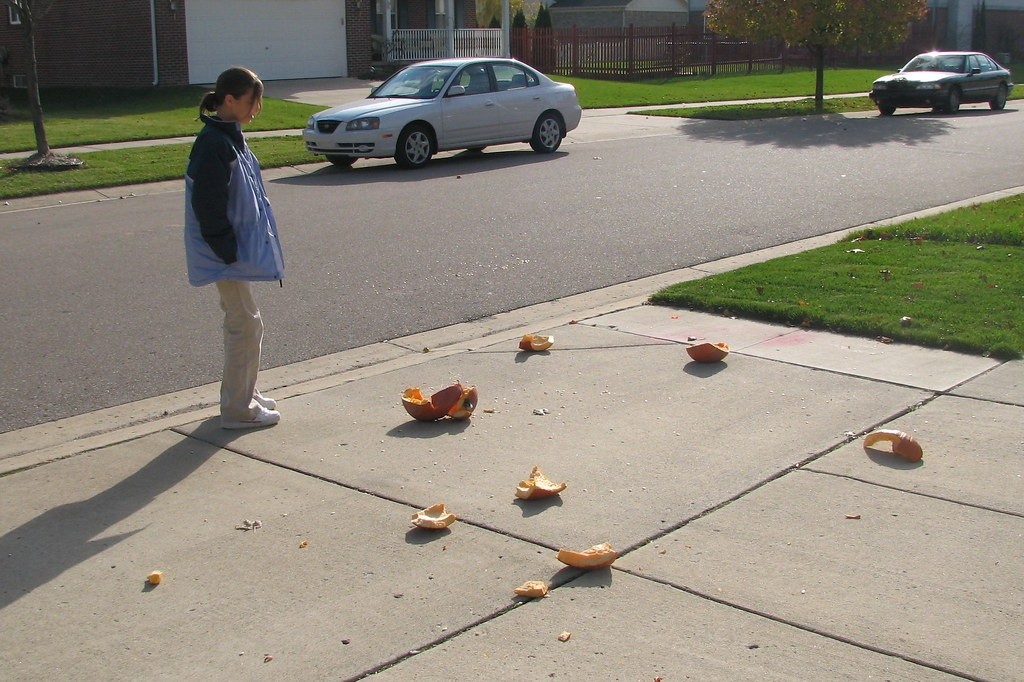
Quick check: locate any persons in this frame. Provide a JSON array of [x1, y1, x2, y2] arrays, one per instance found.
[[184, 66, 285, 429]]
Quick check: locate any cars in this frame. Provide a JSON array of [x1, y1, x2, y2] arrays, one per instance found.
[[869, 52, 1014, 114], [301, 56, 582, 169]]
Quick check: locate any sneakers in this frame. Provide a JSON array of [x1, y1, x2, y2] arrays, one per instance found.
[[220, 397, 281, 430]]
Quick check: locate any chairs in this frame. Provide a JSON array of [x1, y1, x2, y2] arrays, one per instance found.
[[436, 68, 528, 94]]
